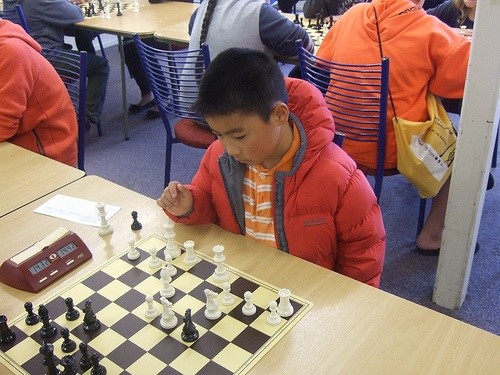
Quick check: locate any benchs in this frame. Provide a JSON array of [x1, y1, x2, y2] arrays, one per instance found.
[[41, 48, 88, 172]]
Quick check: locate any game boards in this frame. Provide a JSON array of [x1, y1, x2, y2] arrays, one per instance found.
[[80, 0, 145, 19], [0, 232, 315, 375], [302, 23, 335, 46]]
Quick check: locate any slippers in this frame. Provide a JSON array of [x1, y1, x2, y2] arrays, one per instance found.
[[416, 241, 479, 256]]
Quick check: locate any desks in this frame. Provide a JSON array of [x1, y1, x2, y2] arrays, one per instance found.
[[71, 0, 200, 141], [0, 141, 85, 217], [0, 175, 500, 375], [154, 13, 473, 118]]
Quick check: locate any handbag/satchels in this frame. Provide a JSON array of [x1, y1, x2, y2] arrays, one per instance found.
[[392, 92, 458, 198]]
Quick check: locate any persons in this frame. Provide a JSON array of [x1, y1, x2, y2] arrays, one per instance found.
[[127, 1, 498, 254], [156, 47, 387, 289], [2, 1, 111, 132], [0, 17, 78, 167]]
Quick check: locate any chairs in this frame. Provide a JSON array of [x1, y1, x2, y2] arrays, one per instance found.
[[132, 32, 219, 190], [296, 38, 427, 238]]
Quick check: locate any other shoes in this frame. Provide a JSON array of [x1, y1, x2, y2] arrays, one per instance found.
[[128, 97, 157, 113], [143, 99, 173, 120], [75, 111, 91, 130]]
